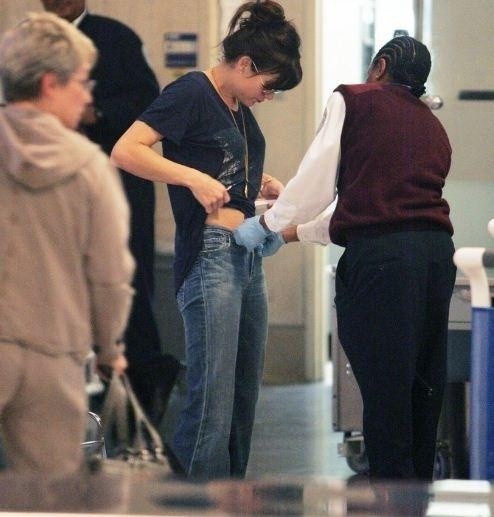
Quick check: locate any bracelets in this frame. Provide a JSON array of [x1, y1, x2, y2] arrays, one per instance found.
[[259, 213, 272, 236], [261, 177, 272, 194]]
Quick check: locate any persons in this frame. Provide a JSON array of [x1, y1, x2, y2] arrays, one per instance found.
[[233, 36, 459, 480], [39, 0, 162, 446], [109, 0, 302, 478], [1, 14, 140, 474]]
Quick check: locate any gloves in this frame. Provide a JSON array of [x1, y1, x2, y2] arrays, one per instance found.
[[232, 213, 271, 252], [255, 230, 288, 258]]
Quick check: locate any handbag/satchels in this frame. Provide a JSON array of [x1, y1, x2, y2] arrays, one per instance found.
[[90, 369, 175, 482]]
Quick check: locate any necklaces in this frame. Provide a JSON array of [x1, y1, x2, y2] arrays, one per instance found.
[[210, 64, 250, 199]]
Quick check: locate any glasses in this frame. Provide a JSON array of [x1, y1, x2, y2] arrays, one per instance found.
[[252, 61, 283, 96]]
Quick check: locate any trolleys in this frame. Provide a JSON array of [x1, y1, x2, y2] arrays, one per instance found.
[[323, 262, 494, 478]]
